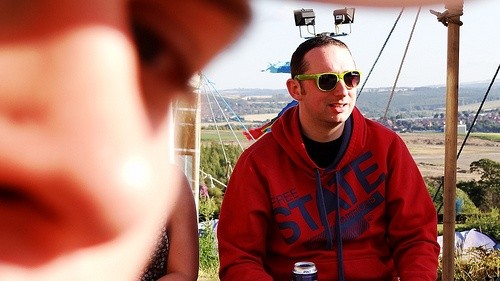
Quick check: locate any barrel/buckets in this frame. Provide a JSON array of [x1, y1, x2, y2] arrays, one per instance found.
[[456, 199, 463, 216]]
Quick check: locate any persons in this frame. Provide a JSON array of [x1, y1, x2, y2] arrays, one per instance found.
[[139, 170, 199, 281], [217, 34, 441, 281], [0, 0, 250, 281]]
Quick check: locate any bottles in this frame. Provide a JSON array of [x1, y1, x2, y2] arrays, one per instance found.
[[292, 262, 317, 281]]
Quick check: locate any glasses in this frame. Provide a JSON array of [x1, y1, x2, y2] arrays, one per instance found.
[[291, 70, 364, 92]]
